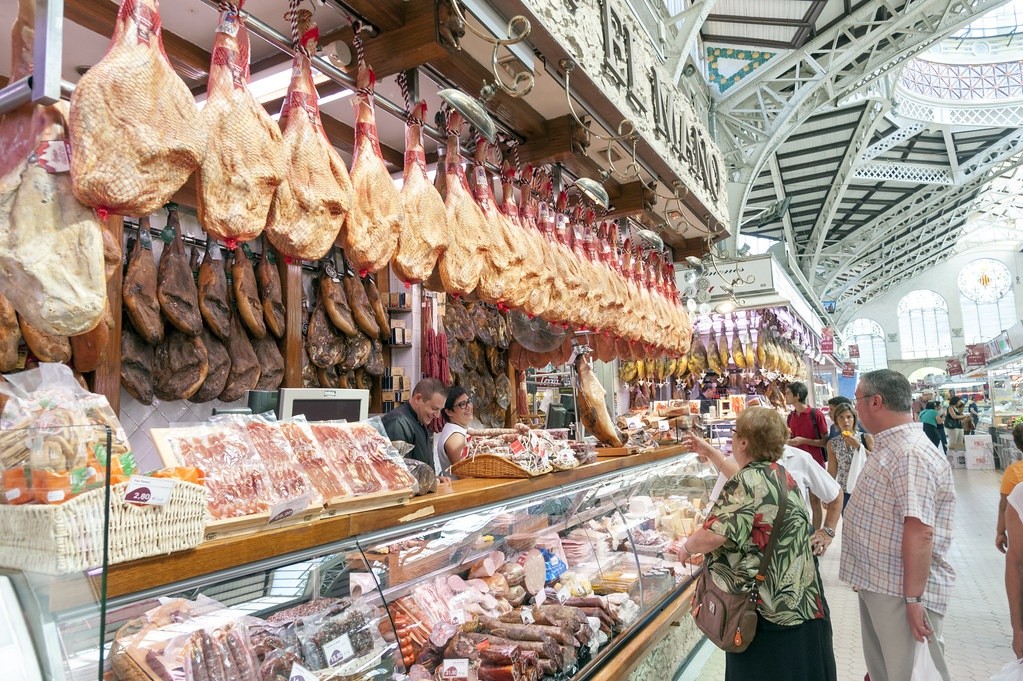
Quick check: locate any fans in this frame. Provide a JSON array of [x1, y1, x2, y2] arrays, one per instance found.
[[508, 307, 567, 353]]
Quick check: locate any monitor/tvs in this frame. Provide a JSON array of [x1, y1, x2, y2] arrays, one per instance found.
[[545, 402, 567, 429], [821, 300, 837, 314], [757, 196, 793, 228], [275, 388, 370, 424]]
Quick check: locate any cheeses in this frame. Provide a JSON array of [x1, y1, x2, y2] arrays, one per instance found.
[[626, 497, 707, 538]]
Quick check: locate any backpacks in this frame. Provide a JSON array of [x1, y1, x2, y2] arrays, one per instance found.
[[811, 407, 828, 462]]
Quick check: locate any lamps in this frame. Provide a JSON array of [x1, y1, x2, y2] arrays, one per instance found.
[[194, 40, 350, 110], [703, 213, 756, 306], [784, 307, 825, 364], [673, 180, 730, 275], [559, 60, 642, 211], [436, 0, 534, 144], [630, 134, 689, 252]]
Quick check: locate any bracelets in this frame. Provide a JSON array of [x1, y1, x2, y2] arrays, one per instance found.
[[717, 456, 728, 471], [904, 596, 921, 603], [683, 544, 691, 555]]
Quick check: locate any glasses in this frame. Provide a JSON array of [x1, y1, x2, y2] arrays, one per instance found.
[[853, 393, 886, 405], [729, 424, 740, 436], [453, 397, 472, 410]]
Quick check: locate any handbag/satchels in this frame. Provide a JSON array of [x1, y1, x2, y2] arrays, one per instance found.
[[989, 658, 1023, 681], [846, 444, 868, 494], [688, 566, 759, 653], [946, 407, 960, 428], [911, 636, 943, 681]]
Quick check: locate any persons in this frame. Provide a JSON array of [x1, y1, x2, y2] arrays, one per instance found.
[[678, 369, 1023, 681], [379, 377, 474, 483]]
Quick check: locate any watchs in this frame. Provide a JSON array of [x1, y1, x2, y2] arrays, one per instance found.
[[822, 526, 835, 538]]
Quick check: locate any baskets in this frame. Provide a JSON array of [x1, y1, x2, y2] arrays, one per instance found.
[[0, 477, 211, 576]]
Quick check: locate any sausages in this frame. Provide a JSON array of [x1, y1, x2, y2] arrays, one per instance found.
[[379, 597, 623, 681]]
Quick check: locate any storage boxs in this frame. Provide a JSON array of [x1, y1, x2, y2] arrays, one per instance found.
[[947, 432, 995, 469]]
